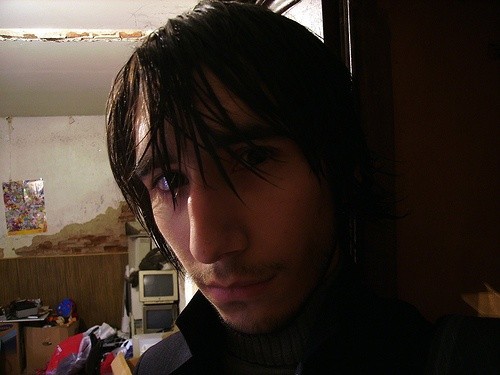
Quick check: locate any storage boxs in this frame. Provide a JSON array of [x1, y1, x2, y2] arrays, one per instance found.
[[24, 318, 80, 375]]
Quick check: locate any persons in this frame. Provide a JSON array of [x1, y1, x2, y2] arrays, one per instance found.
[[105, 0, 500, 375]]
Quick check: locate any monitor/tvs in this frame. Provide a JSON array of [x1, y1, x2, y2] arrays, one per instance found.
[[139, 270, 178, 304], [143, 305, 177, 334]]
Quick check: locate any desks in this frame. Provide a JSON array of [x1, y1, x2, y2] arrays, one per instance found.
[[0, 311, 53, 370]]
